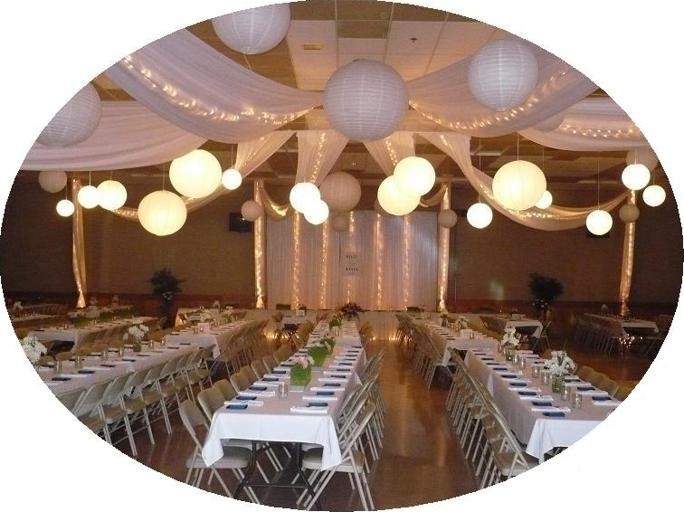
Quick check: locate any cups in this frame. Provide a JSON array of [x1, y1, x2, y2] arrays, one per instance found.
[[75, 355, 81, 368], [148, 339, 154, 348], [532, 366, 539, 376], [541, 372, 549, 383], [571, 392, 582, 408], [64, 323, 69, 329], [118, 345, 125, 357], [161, 337, 167, 345], [101, 351, 107, 360], [561, 386, 570, 399], [277, 383, 288, 399], [519, 358, 526, 369], [54, 360, 62, 372], [513, 354, 519, 363]]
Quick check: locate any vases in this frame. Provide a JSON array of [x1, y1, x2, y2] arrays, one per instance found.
[[133, 338, 142, 352]]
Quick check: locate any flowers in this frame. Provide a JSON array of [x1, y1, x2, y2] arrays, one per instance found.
[[127, 324, 150, 339]]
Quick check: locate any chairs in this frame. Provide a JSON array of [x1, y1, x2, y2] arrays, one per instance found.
[[78, 380, 112, 444], [133, 365, 158, 448], [151, 361, 171, 436], [236, 366, 258, 385], [214, 379, 285, 470], [251, 360, 268, 378], [199, 388, 279, 484], [572, 312, 619, 355], [273, 350, 285, 364], [390, 309, 460, 389], [209, 316, 272, 377], [179, 400, 256, 505], [575, 365, 592, 382], [200, 344, 218, 388], [588, 372, 606, 389], [598, 380, 618, 398], [181, 343, 204, 405], [59, 385, 81, 410], [107, 372, 137, 456], [293, 353, 394, 511], [444, 357, 530, 490], [279, 345, 294, 358], [230, 373, 247, 392], [263, 356, 279, 372], [165, 352, 186, 429]]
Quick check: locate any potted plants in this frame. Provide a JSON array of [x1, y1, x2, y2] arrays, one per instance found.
[[147, 270, 181, 330], [343, 303, 360, 323], [528, 272, 564, 345], [329, 312, 343, 336], [34, 340, 200, 397]]
